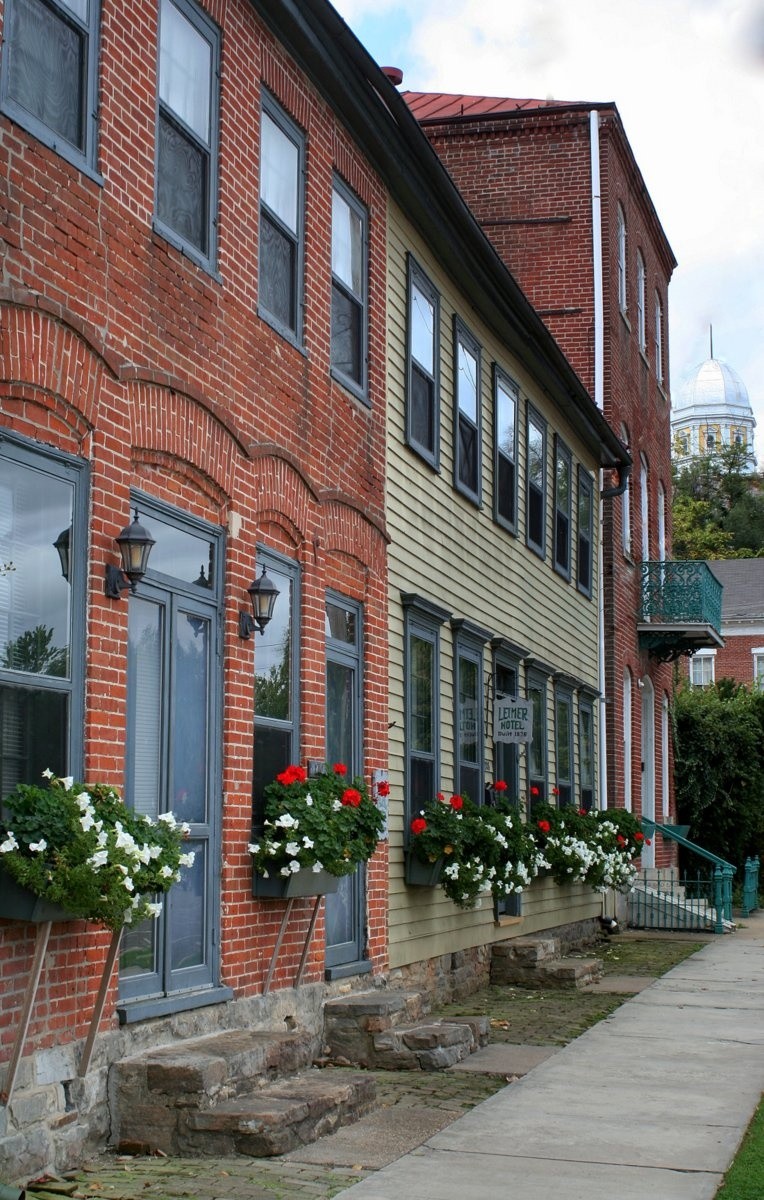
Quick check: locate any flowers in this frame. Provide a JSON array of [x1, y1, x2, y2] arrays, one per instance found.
[[412, 773, 648, 911], [4, 765, 201, 927], [245, 760, 391, 880]]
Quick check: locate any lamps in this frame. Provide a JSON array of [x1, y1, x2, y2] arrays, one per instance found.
[[105, 503, 161, 599], [239, 561, 278, 639], [186, 562, 210, 636], [51, 524, 69, 587]]
